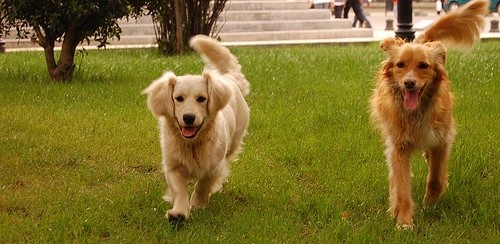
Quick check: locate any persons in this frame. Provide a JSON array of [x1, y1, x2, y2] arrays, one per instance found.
[[313, 0, 373, 29]]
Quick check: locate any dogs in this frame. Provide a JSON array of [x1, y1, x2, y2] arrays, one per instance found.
[[141, 33, 253, 225], [367, 1, 489, 232]]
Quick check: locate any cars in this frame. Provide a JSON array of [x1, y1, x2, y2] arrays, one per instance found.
[[443, 0, 500, 15]]
